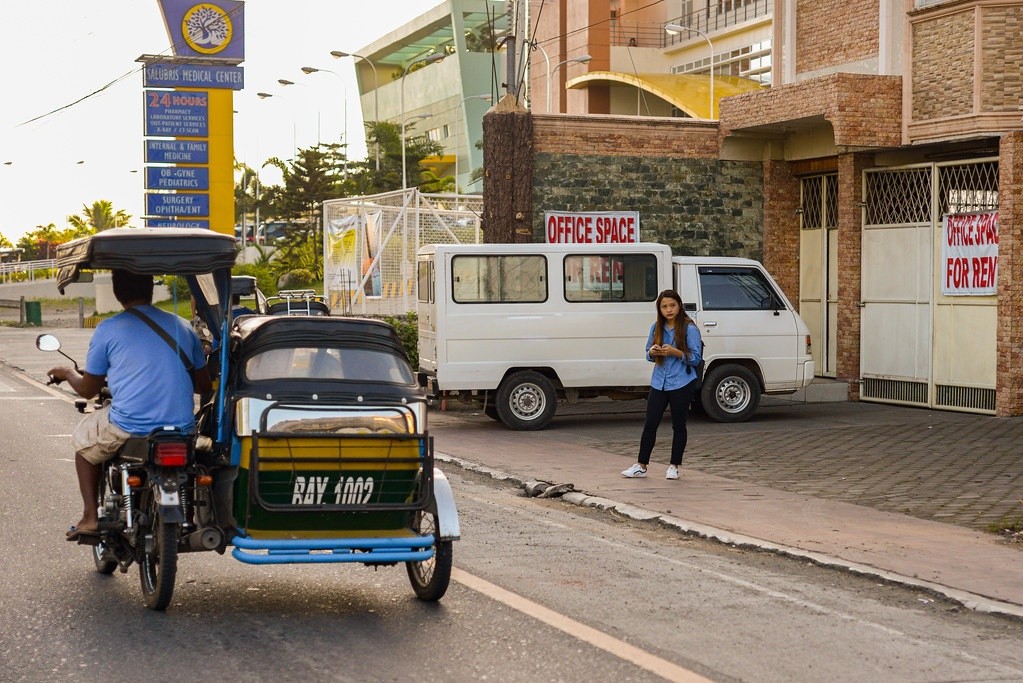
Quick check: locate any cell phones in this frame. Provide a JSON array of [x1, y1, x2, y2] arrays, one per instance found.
[[652, 349, 657, 350]]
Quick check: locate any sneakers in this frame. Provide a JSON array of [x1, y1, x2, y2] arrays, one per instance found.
[[621, 463, 647, 478], [666, 465, 680, 480]]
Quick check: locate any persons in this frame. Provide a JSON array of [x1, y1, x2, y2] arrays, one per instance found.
[[190, 290, 246, 319], [46, 268, 211, 542], [622, 289, 701, 478]]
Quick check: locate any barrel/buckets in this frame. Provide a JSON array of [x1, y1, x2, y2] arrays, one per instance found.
[[25, 302, 42, 326]]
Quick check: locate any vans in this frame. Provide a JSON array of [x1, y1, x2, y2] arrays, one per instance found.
[[416, 242, 817, 429]]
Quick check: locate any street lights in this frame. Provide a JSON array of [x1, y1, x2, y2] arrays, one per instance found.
[[661, 22, 721, 123], [277, 79, 323, 159], [327, 49, 380, 173], [256, 92, 297, 171], [303, 67, 347, 183], [495, 37, 551, 114], [402, 52, 448, 311], [453, 93, 494, 210], [550, 54, 593, 113]]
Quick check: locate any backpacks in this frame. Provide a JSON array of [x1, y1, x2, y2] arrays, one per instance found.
[[683, 320, 705, 389]]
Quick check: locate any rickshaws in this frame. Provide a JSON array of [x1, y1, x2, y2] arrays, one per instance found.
[[35, 223, 460, 612]]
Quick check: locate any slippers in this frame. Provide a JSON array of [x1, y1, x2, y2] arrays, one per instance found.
[[65, 526, 100, 541]]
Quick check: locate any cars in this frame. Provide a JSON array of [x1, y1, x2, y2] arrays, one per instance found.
[[235, 220, 306, 245]]
